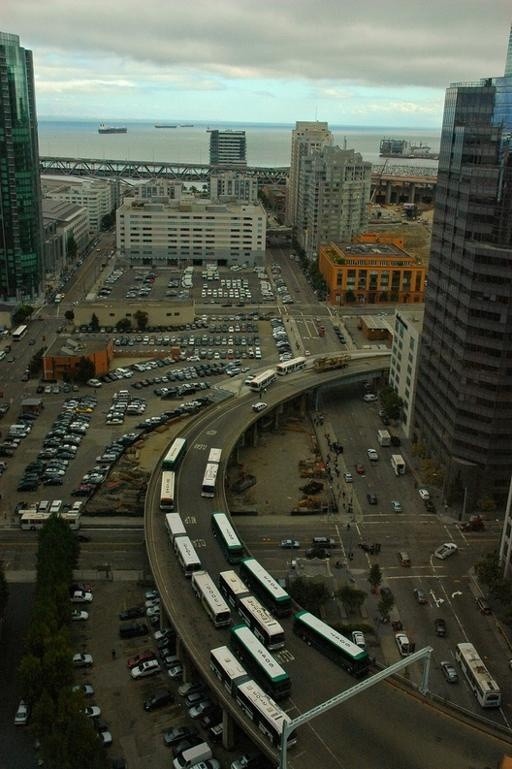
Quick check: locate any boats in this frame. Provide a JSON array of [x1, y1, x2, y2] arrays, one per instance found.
[[98, 122, 127, 134]]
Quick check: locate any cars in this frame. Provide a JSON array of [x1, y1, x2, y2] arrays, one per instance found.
[[0, 264, 503, 769]]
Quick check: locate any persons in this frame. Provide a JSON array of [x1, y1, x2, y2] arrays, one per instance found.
[[315, 414, 357, 532], [111, 648, 117, 661]]
